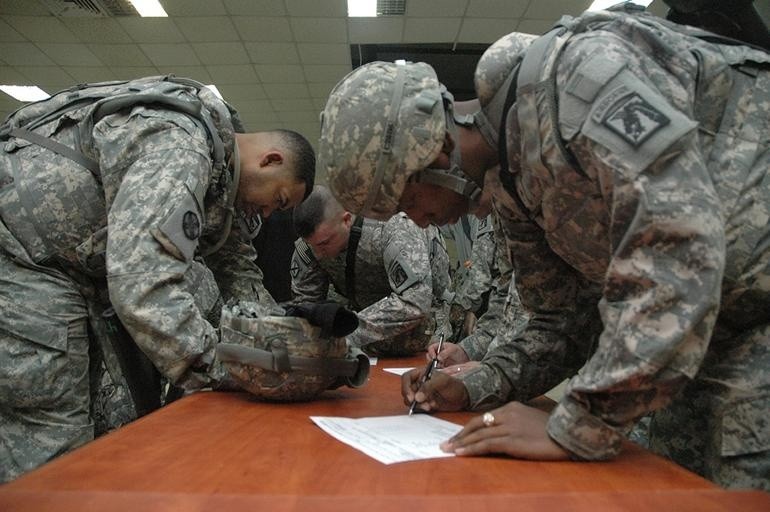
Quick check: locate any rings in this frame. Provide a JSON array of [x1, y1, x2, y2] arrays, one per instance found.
[[483, 410, 495, 426]]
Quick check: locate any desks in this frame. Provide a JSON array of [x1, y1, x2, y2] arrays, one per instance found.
[[0, 349, 769, 511]]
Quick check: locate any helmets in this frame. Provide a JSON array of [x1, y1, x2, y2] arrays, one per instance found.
[[317, 58, 449, 224], [216, 296, 371, 404]]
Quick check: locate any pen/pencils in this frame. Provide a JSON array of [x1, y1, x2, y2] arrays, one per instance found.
[[408, 359, 436, 417], [437, 332, 445, 354]]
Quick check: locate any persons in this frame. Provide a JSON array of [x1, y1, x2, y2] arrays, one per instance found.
[[320, 2, 770, 512], [292, 184, 531, 378], [1, 74, 313, 484]]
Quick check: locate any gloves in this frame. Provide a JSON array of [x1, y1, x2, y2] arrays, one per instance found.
[[281, 300, 361, 342]]
